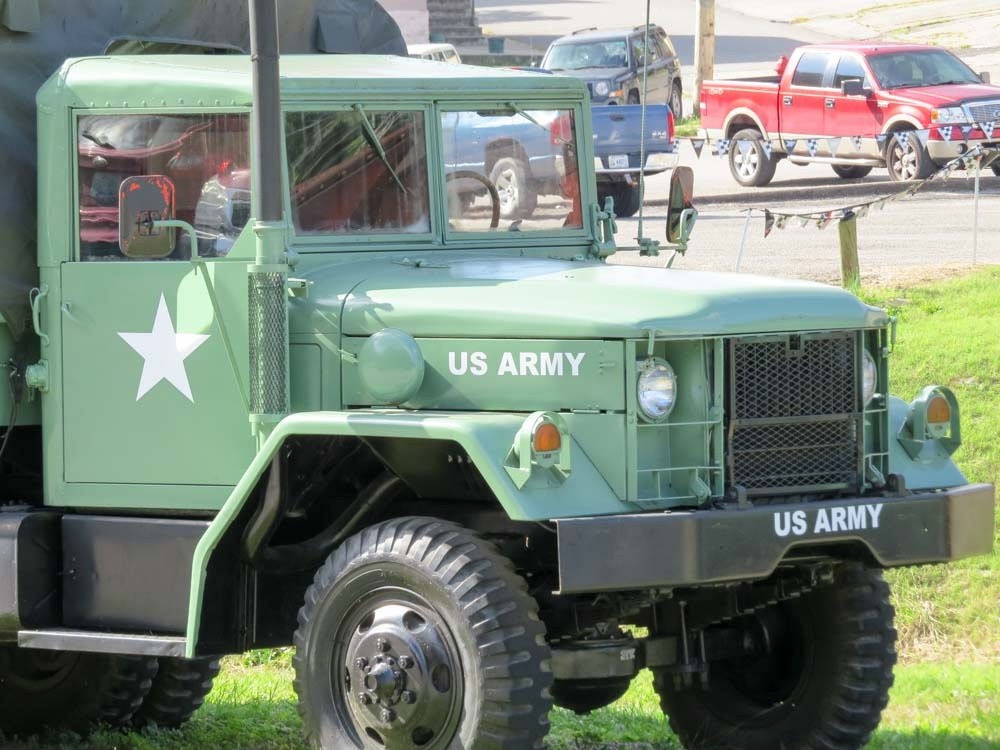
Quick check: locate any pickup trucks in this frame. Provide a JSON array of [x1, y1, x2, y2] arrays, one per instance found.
[[698, 42, 1000, 187], [435, 66, 679, 224]]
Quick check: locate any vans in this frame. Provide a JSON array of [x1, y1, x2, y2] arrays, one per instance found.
[[405, 43, 463, 66]]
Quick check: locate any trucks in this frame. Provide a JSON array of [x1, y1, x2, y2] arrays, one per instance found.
[[2, 0, 1000, 750]]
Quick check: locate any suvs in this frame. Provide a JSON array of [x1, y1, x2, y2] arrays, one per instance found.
[[538, 24, 685, 123]]
